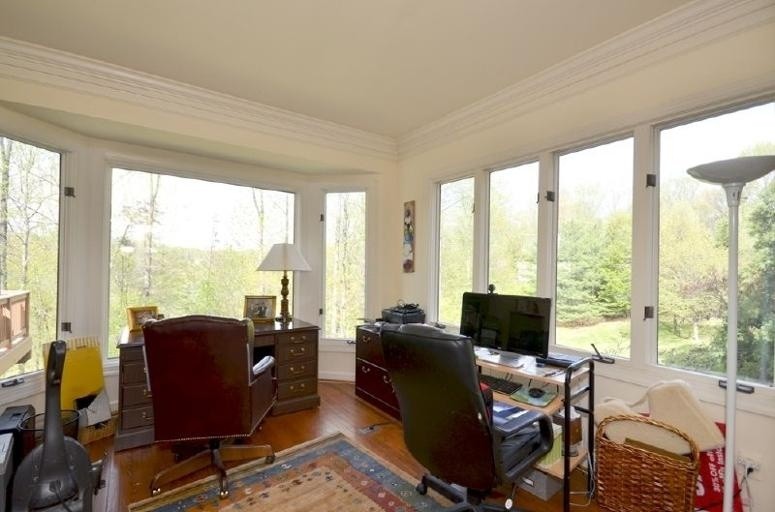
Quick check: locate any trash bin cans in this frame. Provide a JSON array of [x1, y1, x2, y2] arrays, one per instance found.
[[16, 409, 81, 463]]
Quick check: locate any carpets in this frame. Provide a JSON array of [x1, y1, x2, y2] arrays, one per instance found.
[[125, 429, 473, 511]]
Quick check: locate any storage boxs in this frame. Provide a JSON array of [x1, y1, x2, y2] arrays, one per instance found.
[[514, 466, 564, 502]]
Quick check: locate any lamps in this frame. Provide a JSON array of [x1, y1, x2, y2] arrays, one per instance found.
[[686, 153, 775, 512], [255, 242, 313, 322]]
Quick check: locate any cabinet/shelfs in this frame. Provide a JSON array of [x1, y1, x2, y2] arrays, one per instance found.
[[353, 324, 403, 424]]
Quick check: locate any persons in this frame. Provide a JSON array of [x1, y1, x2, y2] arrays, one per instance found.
[[246, 302, 272, 318]]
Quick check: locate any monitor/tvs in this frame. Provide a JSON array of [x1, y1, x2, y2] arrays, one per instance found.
[[460, 292, 551, 368]]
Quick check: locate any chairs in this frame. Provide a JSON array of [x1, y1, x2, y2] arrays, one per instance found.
[[140, 314, 276, 500], [378, 325, 553, 511]]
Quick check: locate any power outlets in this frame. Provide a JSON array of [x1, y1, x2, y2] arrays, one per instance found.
[[735, 455, 763, 481]]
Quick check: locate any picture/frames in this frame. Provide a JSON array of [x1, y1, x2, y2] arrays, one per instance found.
[[244, 294, 277, 324], [128, 307, 158, 332]]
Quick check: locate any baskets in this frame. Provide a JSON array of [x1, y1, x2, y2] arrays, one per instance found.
[[589, 403, 699, 512]]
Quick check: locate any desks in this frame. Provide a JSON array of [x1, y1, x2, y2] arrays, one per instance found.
[[111, 317, 322, 451], [467, 345, 598, 512]]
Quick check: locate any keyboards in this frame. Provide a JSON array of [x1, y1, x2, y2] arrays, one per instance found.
[[479, 374, 522, 396]]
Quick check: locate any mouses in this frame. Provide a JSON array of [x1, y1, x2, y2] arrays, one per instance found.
[[529, 388, 542, 397]]
